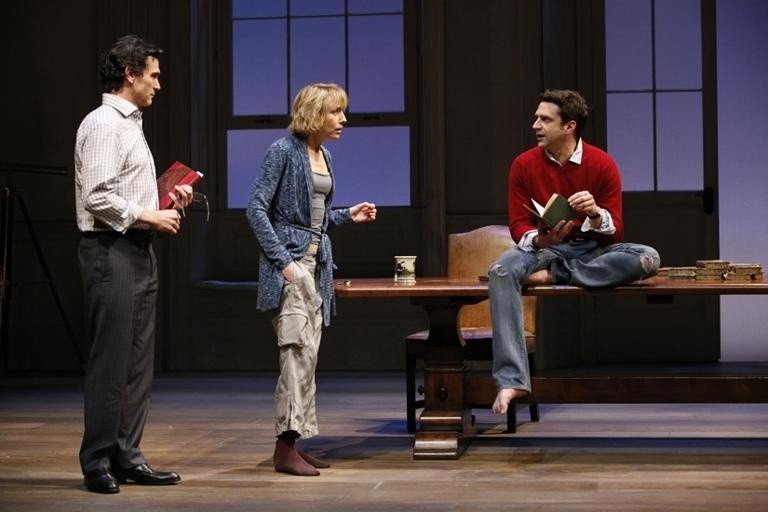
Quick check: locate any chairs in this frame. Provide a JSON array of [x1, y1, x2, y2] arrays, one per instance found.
[[407, 224, 539, 433]]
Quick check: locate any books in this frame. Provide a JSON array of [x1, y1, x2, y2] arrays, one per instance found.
[[521, 193, 577, 228], [657, 259, 765, 281], [156, 160, 205, 210]]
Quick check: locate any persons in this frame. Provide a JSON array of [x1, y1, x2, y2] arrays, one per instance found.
[[72, 32, 194, 493], [245, 81, 378, 479], [486, 86, 661, 416]]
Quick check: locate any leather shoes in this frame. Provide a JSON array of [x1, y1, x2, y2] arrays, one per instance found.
[[112, 458, 182, 486], [83, 471, 121, 494]]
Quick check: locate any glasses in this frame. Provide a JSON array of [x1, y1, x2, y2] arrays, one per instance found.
[[175, 190, 212, 222]]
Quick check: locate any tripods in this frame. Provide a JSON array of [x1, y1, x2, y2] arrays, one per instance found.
[[0, 162, 84, 367]]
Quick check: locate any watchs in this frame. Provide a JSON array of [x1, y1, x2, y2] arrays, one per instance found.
[[587, 211, 603, 220]]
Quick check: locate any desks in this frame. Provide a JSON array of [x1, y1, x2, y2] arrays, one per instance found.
[[332, 275, 768, 434]]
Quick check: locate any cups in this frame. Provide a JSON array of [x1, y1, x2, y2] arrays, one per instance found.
[[393, 253, 417, 284]]
